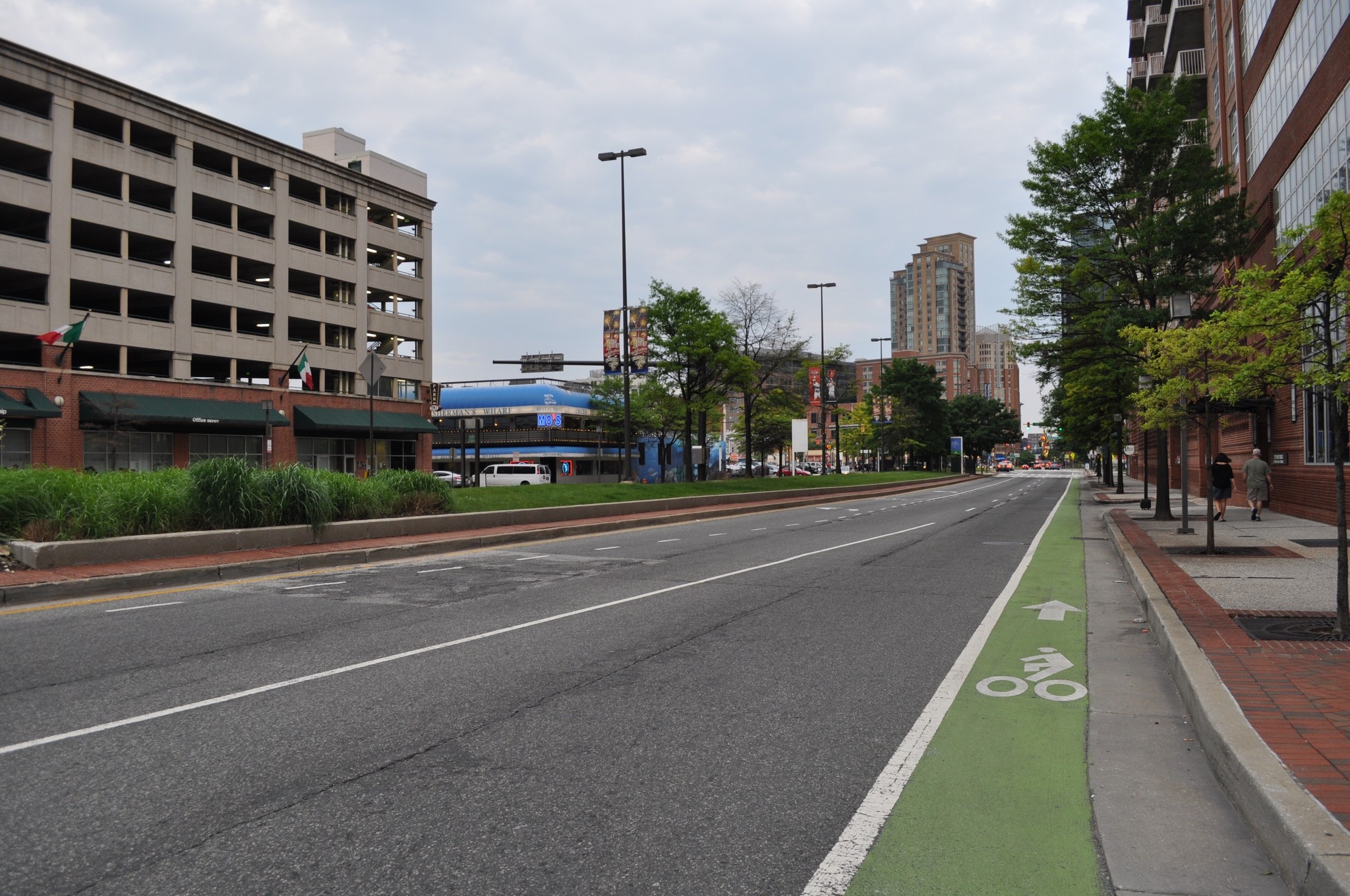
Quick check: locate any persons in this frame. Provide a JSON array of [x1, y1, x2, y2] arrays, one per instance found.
[[826, 378, 837, 401], [826, 460, 836, 475], [1209, 453, 1237, 522], [808, 464, 813, 476], [1239, 448, 1274, 522], [811, 379, 821, 402]]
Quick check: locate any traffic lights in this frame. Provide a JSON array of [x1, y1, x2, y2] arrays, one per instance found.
[[1027, 422, 1030, 431]]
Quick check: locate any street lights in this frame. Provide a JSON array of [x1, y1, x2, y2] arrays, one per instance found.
[[599, 148, 648, 486], [1113, 411, 1125, 494], [871, 338, 892, 472], [1168, 290, 1194, 533], [1139, 372, 1152, 510], [807, 282, 838, 474]]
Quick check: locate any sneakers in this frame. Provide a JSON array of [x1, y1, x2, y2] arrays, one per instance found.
[[1251, 508, 1257, 520], [1255, 516, 1261, 521]]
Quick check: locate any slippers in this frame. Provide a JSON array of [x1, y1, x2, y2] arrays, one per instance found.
[[1213, 511, 1221, 521], [1220, 519, 1226, 522]]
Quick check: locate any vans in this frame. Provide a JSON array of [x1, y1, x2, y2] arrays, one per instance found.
[[472, 464, 551, 487]]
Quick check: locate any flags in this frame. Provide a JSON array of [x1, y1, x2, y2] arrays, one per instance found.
[[34, 317, 85, 346], [297, 352, 314, 391]]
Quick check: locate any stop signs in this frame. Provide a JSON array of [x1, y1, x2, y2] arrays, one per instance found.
[[826, 453, 829, 458]]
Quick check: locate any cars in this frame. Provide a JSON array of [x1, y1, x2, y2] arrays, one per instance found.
[[726, 460, 854, 477], [994, 460, 1060, 472], [433, 470, 470, 488]]
